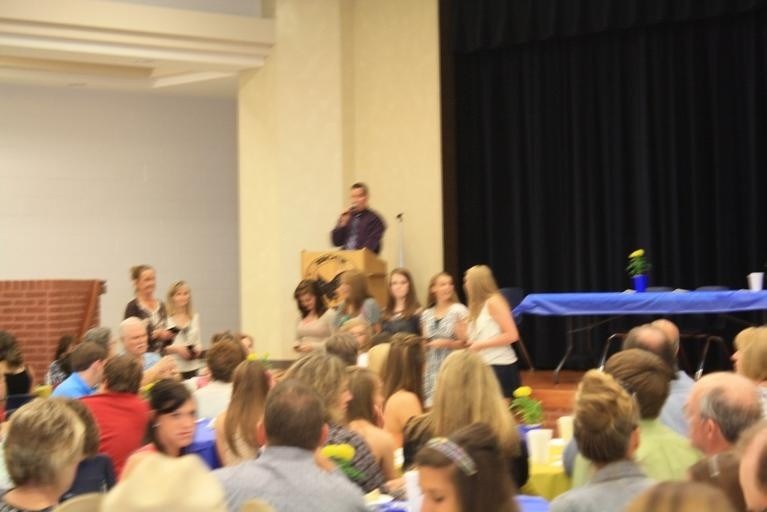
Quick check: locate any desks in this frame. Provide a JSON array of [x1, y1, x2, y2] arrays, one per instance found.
[[183, 416, 218, 470], [517, 441, 572, 503], [378, 490, 549, 512], [512, 288, 767, 386]]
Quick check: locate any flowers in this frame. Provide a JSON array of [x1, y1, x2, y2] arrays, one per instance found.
[[317, 441, 368, 484], [625, 248, 652, 276], [506, 385, 545, 427]]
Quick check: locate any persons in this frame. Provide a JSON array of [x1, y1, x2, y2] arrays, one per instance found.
[[332, 183, 385, 254], [0, 264, 766, 509]]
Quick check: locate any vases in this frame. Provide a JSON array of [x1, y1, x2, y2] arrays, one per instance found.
[[631, 276, 648, 293]]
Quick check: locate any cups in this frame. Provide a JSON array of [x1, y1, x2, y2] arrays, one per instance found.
[[556, 415, 573, 440], [527, 429, 553, 464], [746, 272, 765, 291]]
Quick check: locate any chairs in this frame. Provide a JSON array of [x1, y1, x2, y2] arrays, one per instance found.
[[596, 285, 767, 382]]
